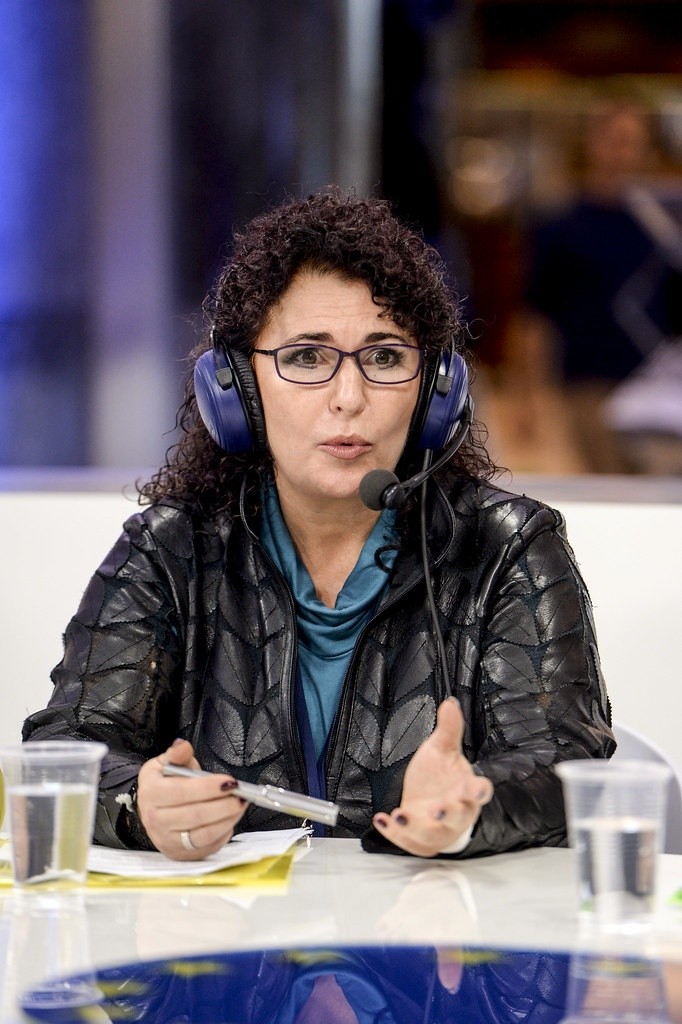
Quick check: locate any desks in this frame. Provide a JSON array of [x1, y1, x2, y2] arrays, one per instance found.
[[0, 836, 682, 1024]]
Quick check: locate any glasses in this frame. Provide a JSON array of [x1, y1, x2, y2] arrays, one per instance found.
[[247, 343, 432, 385]]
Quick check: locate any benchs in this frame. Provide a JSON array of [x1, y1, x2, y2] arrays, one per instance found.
[[0, 493, 679, 843]]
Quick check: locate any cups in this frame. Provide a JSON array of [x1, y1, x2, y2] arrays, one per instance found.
[[561, 922, 677, 1024], [3, 889, 104, 1009], [555, 758, 672, 921], [0, 740, 108, 886]]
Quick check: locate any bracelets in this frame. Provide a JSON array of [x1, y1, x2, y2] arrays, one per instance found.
[[115, 779, 154, 851]]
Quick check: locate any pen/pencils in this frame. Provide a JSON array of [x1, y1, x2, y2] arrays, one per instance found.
[[161, 761, 341, 826]]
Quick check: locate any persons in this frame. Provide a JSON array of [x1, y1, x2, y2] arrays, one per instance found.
[[99, 945, 589, 1024], [23, 193, 618, 862], [544, 94, 682, 381]]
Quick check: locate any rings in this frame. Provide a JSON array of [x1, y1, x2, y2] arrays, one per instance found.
[[180, 831, 197, 851]]
